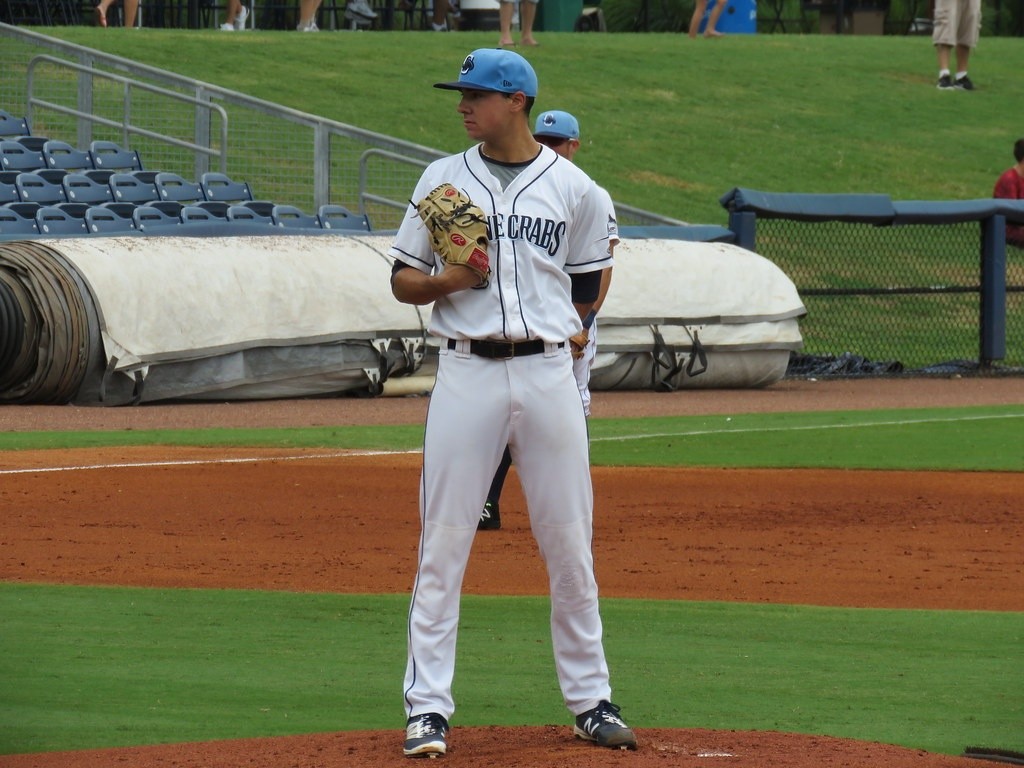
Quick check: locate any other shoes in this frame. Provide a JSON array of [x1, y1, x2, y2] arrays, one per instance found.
[[297, 22, 320, 32], [446, 8, 461, 18], [235, 6, 250, 31], [477, 499, 501, 530], [431, 17, 448, 32], [954, 75, 977, 91], [343, 2, 378, 23], [937, 74, 954, 90], [220, 23, 234, 31]]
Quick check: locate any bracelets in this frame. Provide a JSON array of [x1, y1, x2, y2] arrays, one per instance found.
[[582, 310, 597, 330]]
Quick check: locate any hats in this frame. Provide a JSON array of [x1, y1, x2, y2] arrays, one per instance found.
[[433, 47, 538, 98], [532, 110, 579, 140]]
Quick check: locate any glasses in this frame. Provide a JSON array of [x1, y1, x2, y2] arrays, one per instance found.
[[535, 135, 565, 147]]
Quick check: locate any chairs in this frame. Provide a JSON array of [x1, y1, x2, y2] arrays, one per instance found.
[[0, 108, 372, 239]]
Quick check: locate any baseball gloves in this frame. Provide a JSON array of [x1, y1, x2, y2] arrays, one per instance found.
[[415, 183, 490, 283]]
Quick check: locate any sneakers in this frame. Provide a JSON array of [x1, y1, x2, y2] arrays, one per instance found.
[[574, 700, 637, 750], [403, 713, 449, 758]]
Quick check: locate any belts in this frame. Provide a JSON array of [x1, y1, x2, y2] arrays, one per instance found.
[[447, 338, 564, 361]]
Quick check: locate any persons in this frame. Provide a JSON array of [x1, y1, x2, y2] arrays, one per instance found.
[[498, 0, 538, 48], [689, 0, 727, 40], [219, 0, 249, 30], [992, 138, 1024, 250], [94, 0, 138, 27], [296, 0, 323, 32], [474, 112, 621, 533], [387, 47, 636, 756], [932, 0, 982, 91], [343, 0, 464, 32]]
